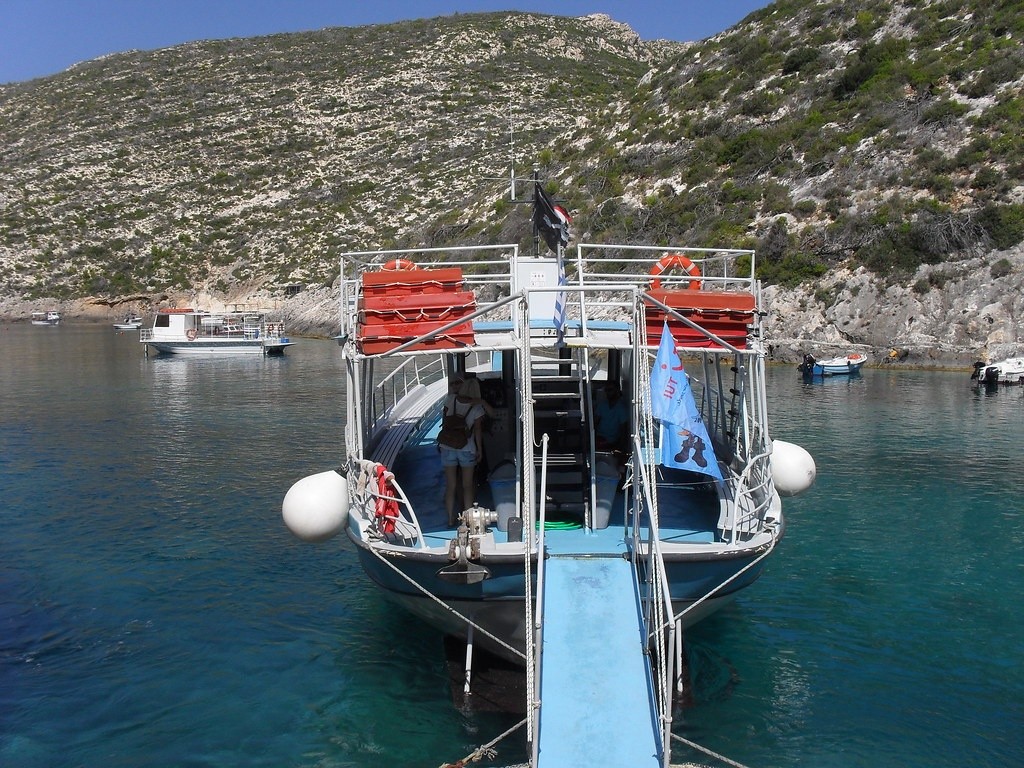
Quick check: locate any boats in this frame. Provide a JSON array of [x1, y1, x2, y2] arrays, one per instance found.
[[112, 316, 142, 329], [971, 354, 1024, 387], [31, 311, 61, 326], [279, 99, 820, 768], [137, 305, 298, 358], [795, 351, 868, 376]]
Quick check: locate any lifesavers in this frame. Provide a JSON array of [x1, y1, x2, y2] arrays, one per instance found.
[[278, 323, 284, 332], [381, 258, 420, 272], [186, 328, 197, 338], [651, 256, 703, 291], [268, 324, 274, 335]]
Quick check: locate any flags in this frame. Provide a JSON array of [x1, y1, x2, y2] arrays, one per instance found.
[[647, 322, 724, 487], [533, 181, 571, 254], [553, 259, 566, 343]]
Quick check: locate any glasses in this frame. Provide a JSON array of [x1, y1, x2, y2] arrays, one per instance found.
[[604, 387, 615, 391], [449, 380, 462, 386]]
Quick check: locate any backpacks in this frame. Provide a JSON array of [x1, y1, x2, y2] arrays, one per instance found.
[[437, 395, 473, 449]]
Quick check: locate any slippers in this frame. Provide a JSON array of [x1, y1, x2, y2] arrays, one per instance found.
[[447, 524, 454, 529]]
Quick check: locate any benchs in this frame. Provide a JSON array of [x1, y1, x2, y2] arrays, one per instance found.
[[360, 359, 757, 542]]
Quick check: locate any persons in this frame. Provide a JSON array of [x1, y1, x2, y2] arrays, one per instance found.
[[594, 381, 628, 449], [436, 373, 496, 528]]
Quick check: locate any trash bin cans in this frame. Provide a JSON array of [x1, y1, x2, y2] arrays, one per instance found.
[[590, 454, 622, 530], [486, 459, 521, 532]]
